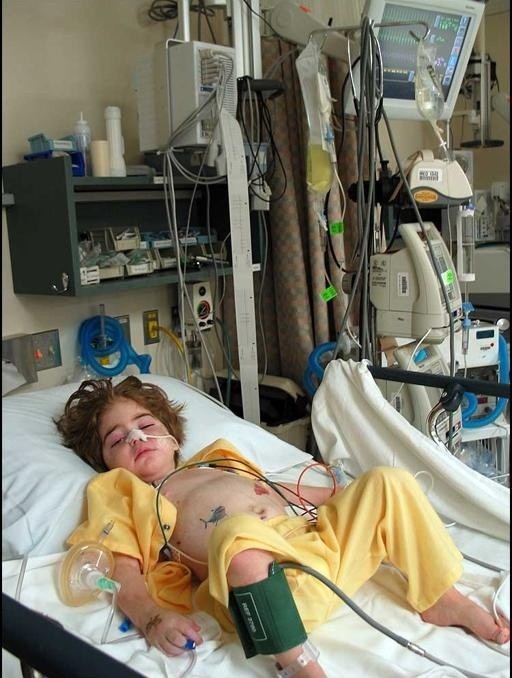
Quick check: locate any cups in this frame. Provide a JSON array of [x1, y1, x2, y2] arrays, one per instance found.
[[91, 140, 111, 178]]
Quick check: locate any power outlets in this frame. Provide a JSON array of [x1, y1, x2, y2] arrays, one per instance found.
[[143, 309, 159, 344]]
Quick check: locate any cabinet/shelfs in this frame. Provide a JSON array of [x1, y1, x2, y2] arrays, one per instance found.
[[0, 156, 267, 297]]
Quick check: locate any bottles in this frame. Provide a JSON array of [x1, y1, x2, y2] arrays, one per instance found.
[[75, 119, 98, 179]]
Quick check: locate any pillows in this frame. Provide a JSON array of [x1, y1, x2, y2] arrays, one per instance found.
[[2, 373, 315, 564]]
